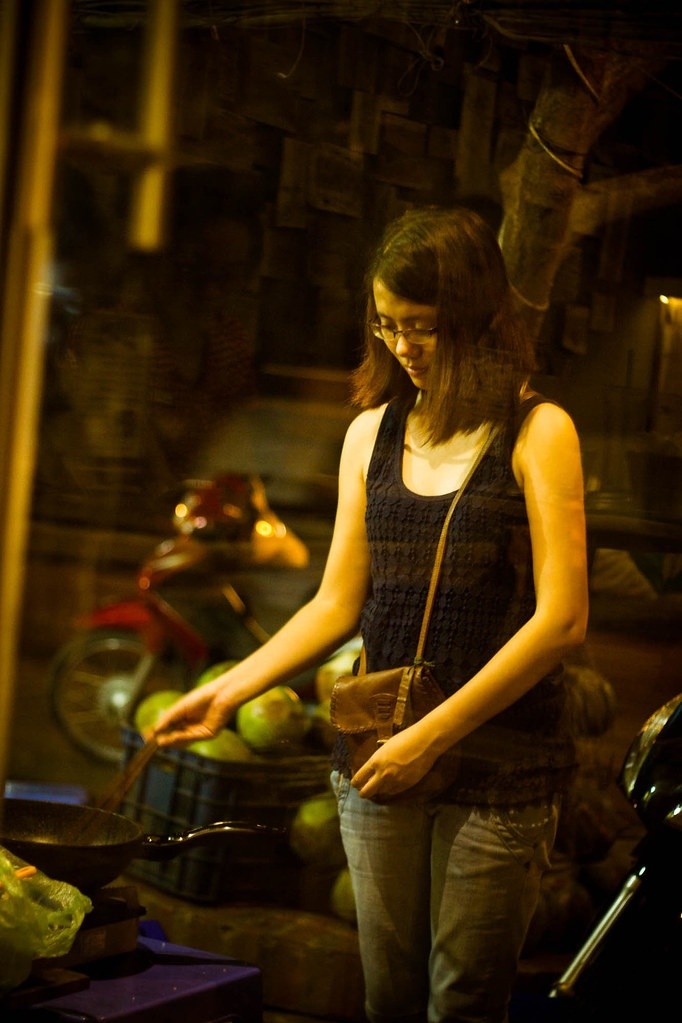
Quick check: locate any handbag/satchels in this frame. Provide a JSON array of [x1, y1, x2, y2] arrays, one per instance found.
[[330, 661, 464, 803]]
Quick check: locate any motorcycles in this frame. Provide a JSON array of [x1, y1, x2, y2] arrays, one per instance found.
[[52, 470, 324, 768]]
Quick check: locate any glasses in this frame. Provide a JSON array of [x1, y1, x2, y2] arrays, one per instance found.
[[368, 316, 438, 345]]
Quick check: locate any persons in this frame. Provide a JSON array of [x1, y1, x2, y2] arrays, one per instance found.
[[146, 209, 591, 1022]]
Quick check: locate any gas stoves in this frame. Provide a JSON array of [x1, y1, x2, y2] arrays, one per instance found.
[[32, 895, 148, 976]]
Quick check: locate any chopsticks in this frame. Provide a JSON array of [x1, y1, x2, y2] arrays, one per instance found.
[[75, 735, 161, 842]]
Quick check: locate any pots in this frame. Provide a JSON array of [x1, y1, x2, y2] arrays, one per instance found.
[[1, 796, 286, 894]]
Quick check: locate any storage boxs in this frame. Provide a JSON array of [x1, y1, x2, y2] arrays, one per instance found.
[[113, 725, 334, 902]]
